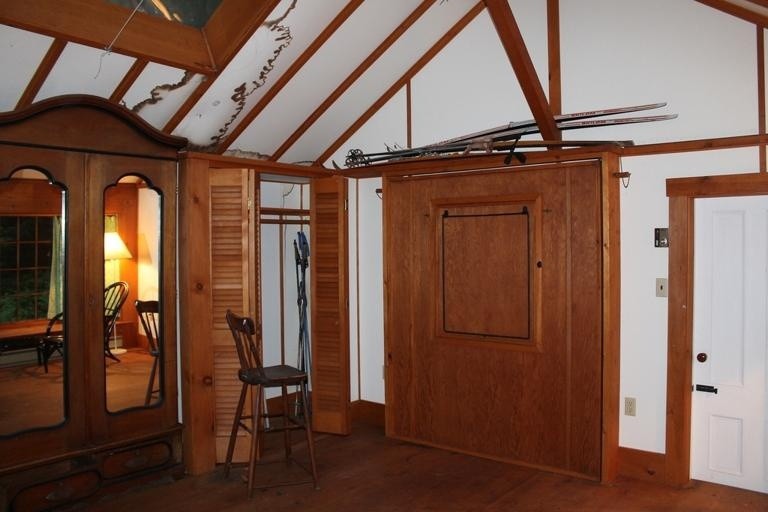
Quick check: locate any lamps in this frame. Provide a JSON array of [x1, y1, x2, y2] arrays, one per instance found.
[[104, 232, 134, 356]]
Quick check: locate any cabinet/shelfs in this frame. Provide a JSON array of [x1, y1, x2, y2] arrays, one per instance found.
[[1, 93, 186, 511], [179, 151, 353, 477]]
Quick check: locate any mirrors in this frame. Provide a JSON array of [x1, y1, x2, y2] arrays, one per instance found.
[[100, 170, 166, 417], [1, 164, 71, 442]]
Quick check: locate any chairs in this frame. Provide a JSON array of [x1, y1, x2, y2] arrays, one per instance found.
[[134, 299, 161, 405], [222, 308, 321, 499], [35, 281, 131, 373]]
